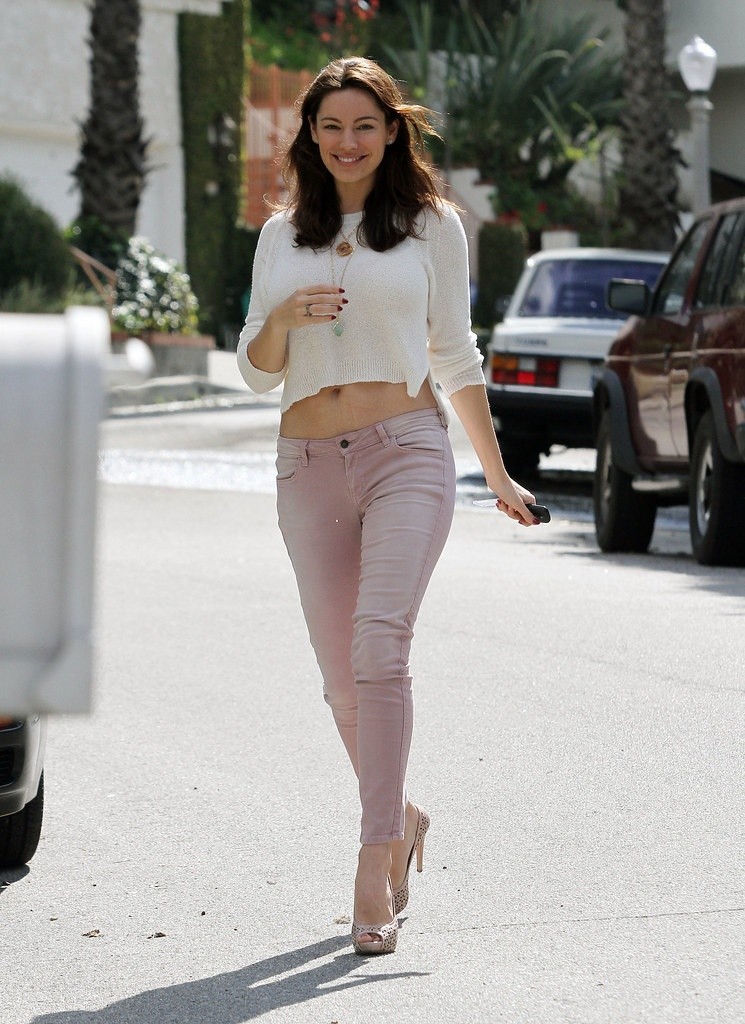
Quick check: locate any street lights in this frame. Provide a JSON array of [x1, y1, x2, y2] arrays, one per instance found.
[[678, 30, 719, 221]]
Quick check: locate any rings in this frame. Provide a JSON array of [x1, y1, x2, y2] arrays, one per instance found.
[[305, 304, 312, 316]]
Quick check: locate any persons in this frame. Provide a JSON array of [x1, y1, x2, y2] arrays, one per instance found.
[[234, 52, 541, 956]]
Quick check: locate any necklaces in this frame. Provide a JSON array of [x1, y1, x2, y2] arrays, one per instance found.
[[329, 217, 364, 337]]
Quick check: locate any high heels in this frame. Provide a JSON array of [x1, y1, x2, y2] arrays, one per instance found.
[[393, 804, 430, 915], [351, 872, 398, 955]]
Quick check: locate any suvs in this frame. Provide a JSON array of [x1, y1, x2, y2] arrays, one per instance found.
[[590, 196, 744, 569], [481, 246, 704, 487]]
